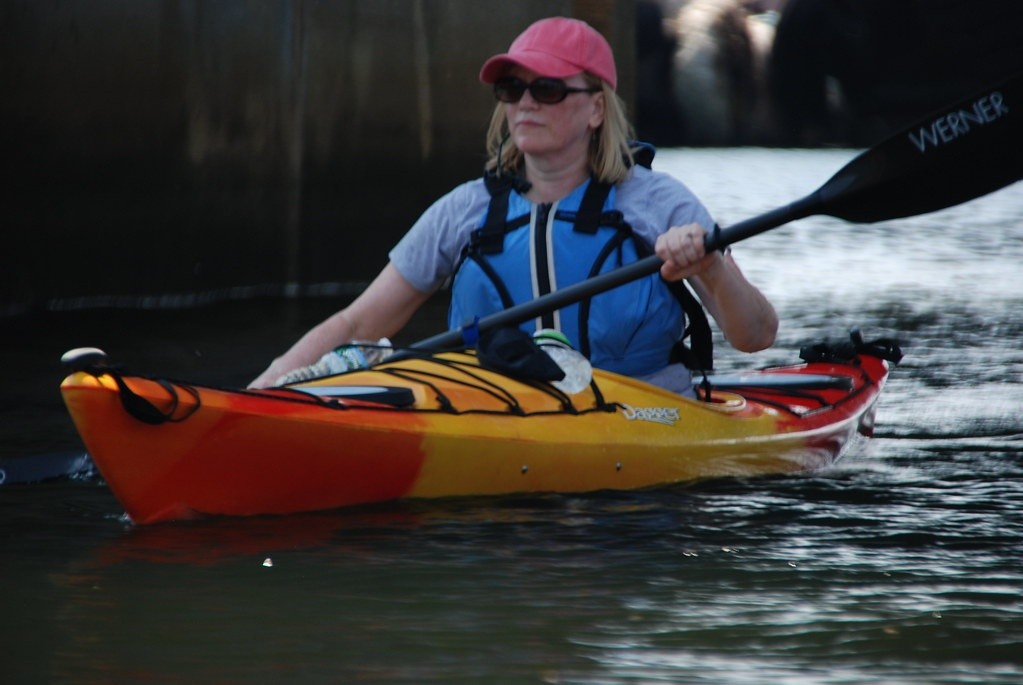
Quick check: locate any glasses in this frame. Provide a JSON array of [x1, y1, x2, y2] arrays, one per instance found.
[[493, 76, 600, 104]]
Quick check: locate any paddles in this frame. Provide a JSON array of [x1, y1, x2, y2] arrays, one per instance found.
[[378, 74, 1023, 356]]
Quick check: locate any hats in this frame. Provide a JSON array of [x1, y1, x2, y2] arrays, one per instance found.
[[479, 16, 617, 96]]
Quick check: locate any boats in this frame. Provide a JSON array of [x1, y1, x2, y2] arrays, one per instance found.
[[57, 329, 902, 525]]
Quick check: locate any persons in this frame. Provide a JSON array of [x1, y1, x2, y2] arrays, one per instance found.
[[247, 16, 778, 402]]
